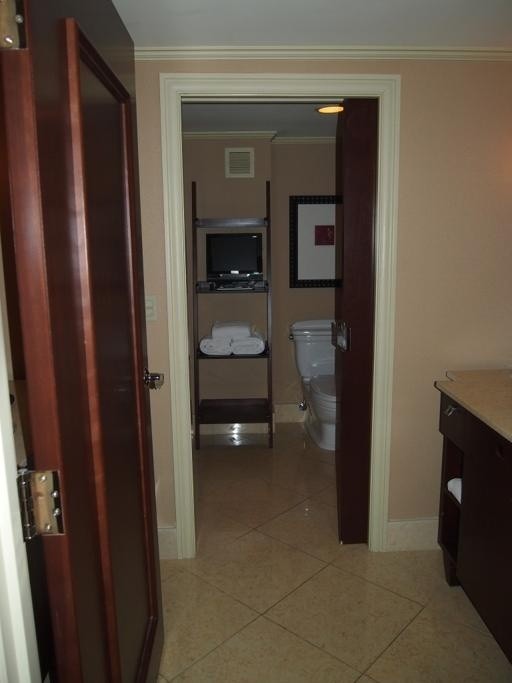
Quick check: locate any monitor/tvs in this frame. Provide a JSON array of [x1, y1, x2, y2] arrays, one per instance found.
[[195, 226, 267, 283]]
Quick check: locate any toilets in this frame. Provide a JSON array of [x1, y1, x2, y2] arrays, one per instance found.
[[289, 320, 336, 451]]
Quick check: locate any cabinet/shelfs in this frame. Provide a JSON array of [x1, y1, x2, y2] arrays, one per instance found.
[[437, 392, 512, 666], [192, 180, 273, 450]]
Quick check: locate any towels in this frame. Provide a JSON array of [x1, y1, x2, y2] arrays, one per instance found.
[[200, 320, 265, 355], [447, 478, 462, 505]]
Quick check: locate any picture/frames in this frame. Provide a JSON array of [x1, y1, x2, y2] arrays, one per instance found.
[[289, 195, 336, 288]]
